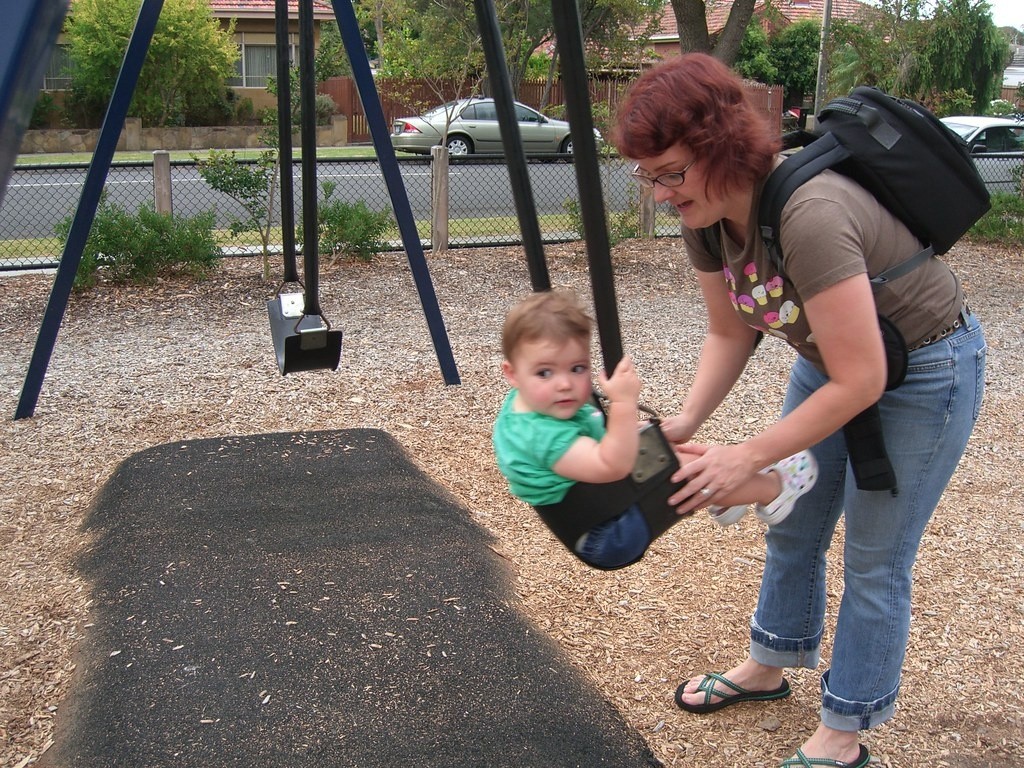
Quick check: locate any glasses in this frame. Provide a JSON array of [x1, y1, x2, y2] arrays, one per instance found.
[[630, 153, 702, 189]]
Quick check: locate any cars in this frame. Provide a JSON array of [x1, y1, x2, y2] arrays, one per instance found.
[[945, 117, 1023, 194], [391, 96, 605, 169]]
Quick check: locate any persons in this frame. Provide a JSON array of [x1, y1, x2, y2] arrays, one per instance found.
[[612, 54, 986, 768], [492, 292, 818, 571]]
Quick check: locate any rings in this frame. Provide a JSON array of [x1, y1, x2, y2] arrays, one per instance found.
[[700, 487, 711, 497]]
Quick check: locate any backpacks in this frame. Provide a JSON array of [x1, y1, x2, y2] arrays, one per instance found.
[[700, 86, 992, 281]]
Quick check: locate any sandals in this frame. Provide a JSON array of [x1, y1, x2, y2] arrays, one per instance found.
[[707, 503, 749, 528], [754, 447, 819, 524]]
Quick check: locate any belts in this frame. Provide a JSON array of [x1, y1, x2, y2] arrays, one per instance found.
[[904, 303, 969, 352]]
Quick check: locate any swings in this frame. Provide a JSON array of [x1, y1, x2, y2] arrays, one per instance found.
[[468, 0, 701, 557], [265, 0, 344, 378]]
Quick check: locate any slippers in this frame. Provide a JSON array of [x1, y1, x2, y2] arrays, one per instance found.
[[674, 671, 791, 714], [779, 742, 870, 768]]
